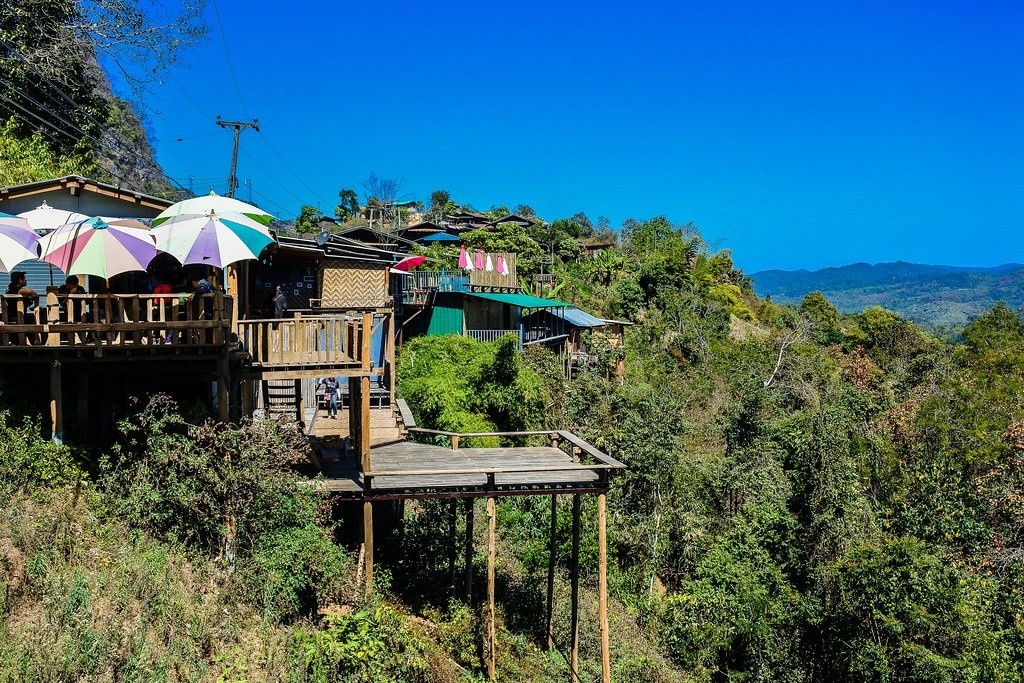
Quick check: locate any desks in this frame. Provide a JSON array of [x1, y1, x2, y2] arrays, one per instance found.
[[30, 307, 65, 344]]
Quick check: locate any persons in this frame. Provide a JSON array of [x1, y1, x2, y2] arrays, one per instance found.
[[4, 272, 216, 343], [319, 377, 342, 420], [272, 285, 288, 318]]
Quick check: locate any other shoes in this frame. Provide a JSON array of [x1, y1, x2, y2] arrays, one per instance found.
[[328, 415, 331, 419], [334, 414, 338, 419], [164, 338, 172, 345]]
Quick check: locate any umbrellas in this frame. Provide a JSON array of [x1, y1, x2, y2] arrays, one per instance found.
[[393, 255, 426, 273], [0, 189, 278, 279], [458, 244, 509, 286]]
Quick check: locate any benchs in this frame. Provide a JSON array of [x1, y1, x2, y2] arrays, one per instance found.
[[0, 294, 235, 347]]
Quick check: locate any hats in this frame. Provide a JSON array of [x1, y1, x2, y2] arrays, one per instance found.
[[10, 271, 27, 279]]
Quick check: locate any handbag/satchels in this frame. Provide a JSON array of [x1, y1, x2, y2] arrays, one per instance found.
[[324, 393, 332, 400]]
